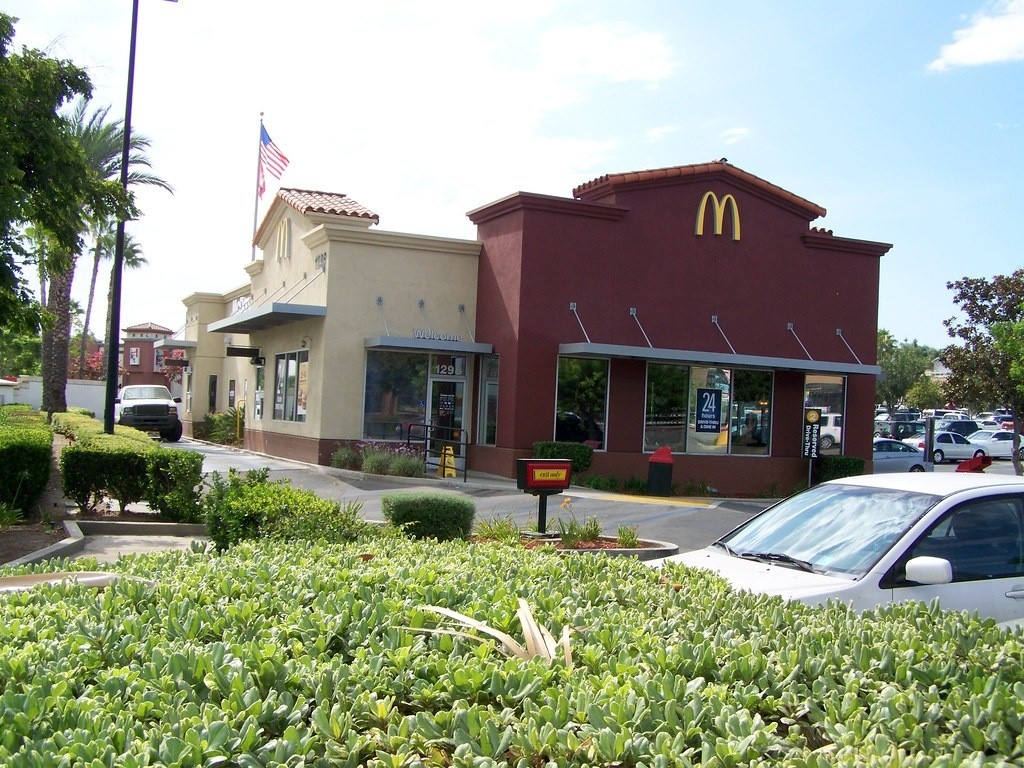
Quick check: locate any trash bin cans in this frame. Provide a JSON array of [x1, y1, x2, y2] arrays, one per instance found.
[[644, 445, 674, 497]]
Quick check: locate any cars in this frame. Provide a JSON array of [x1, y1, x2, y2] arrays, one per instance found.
[[731, 401, 842, 450], [874, 400, 1024, 440], [901, 429, 990, 465], [873, 437, 934, 474], [965, 428, 1024, 461], [637, 472, 1024, 626], [556, 409, 604, 449]]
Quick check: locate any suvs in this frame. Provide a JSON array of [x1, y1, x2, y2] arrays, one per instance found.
[[114, 383, 184, 443]]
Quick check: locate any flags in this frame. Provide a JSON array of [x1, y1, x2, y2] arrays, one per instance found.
[[258, 119, 290, 198]]
